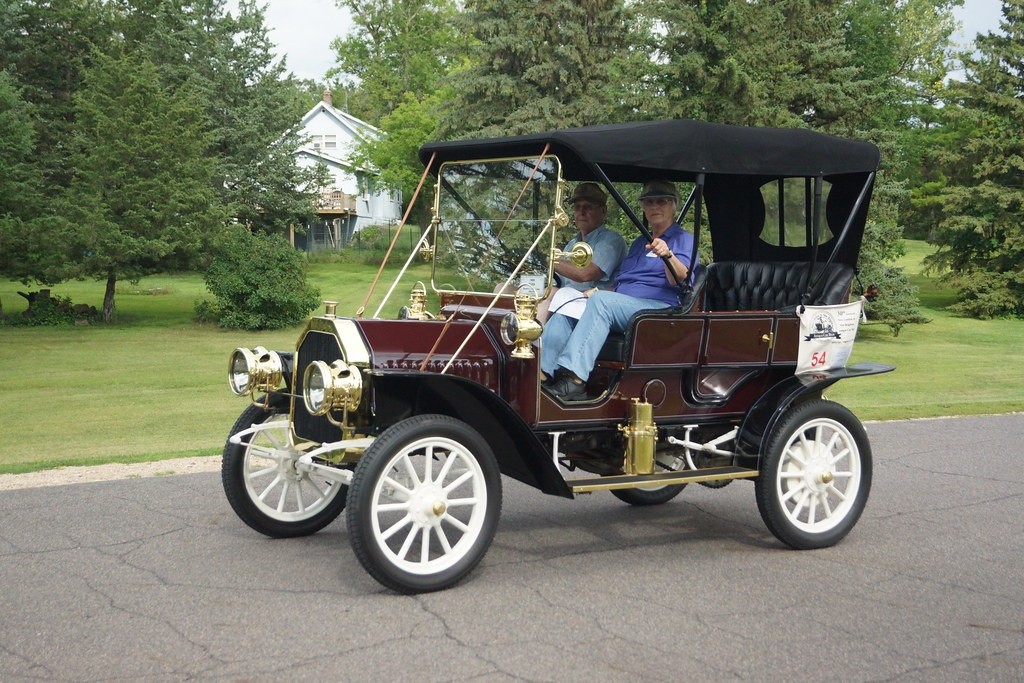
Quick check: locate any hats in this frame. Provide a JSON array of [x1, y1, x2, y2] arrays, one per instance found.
[[638, 178, 679, 200], [566, 183, 607, 204]]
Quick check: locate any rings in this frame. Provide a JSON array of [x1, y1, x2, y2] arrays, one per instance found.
[[660, 248, 664, 251]]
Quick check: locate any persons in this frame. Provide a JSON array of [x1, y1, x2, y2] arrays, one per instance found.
[[493, 182, 626, 323], [541, 180, 700, 400]]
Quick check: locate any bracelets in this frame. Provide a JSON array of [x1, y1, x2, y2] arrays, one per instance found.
[[667, 249, 673, 258]]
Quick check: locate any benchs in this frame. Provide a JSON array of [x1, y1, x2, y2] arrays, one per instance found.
[[595, 265, 709, 368], [696, 260, 855, 310]]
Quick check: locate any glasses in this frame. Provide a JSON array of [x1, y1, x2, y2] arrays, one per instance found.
[[640, 199, 675, 207], [570, 204, 605, 211]]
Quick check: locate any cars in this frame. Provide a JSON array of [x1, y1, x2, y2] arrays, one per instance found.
[[220, 124, 902, 598]]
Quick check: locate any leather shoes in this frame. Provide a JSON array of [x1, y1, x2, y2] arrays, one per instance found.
[[541, 376, 588, 401]]
